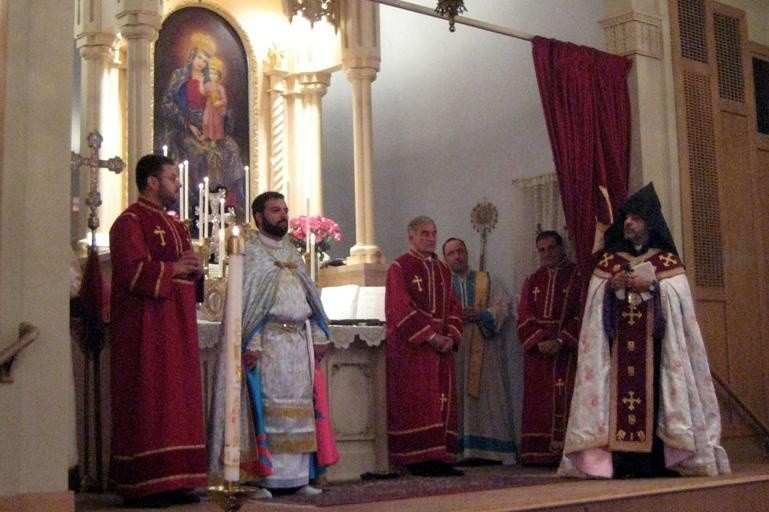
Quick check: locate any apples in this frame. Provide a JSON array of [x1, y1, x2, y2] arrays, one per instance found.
[[179, 158, 241, 280], [304, 191, 318, 282]]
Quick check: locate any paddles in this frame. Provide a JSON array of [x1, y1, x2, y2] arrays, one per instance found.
[[244, 472, 582, 506]]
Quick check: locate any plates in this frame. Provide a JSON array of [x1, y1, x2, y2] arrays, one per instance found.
[[193, 320, 391, 489]]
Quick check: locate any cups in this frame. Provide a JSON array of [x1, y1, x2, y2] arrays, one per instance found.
[[155, 175, 179, 183]]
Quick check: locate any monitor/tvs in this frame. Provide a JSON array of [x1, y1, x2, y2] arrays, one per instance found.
[[627, 260, 658, 302]]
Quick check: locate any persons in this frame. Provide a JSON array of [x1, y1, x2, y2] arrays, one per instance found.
[[161, 32, 246, 206], [197, 55, 228, 150], [549, 182, 735, 482], [440, 236, 521, 469], [205, 189, 335, 503], [103, 151, 214, 511], [515, 230, 588, 468], [384, 215, 471, 479]]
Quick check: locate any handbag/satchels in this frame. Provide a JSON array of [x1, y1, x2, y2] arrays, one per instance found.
[[557, 339, 566, 348], [426, 332, 437, 342]]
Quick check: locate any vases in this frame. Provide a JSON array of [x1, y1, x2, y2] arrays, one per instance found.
[[289, 216, 340, 253]]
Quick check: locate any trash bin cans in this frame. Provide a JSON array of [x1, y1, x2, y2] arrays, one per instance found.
[[648, 278, 657, 298]]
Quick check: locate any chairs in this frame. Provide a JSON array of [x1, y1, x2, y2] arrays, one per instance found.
[[124, 486, 326, 507]]
